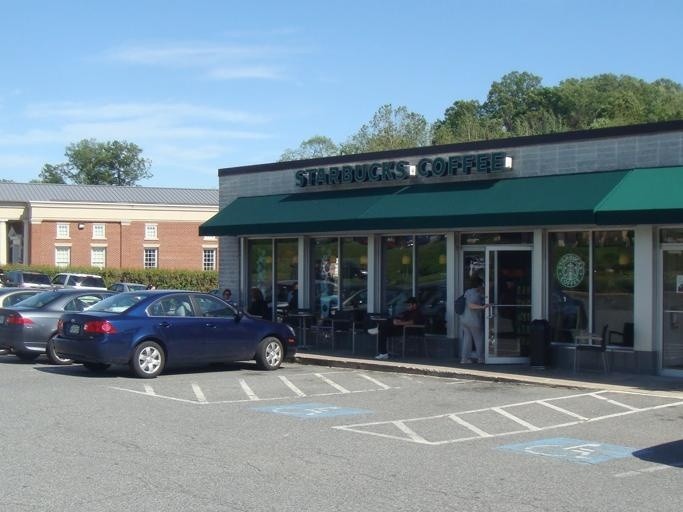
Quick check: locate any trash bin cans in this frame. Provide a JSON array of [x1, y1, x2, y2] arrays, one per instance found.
[[530, 319, 547, 367]]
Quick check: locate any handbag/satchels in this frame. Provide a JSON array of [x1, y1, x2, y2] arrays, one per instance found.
[[455, 295, 465, 314]]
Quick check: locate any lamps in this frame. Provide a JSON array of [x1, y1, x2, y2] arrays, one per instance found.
[[358, 238, 368, 268], [78, 222, 85, 229], [402, 236, 410, 266], [439, 234, 446, 265], [619, 251, 629, 265]]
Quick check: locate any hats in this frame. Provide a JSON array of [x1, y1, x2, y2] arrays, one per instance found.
[[404, 298, 417, 303]]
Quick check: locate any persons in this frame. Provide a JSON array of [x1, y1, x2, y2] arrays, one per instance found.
[[144, 283, 156, 291], [280, 289, 297, 324], [246, 287, 267, 319], [287, 281, 298, 306], [457, 277, 494, 365], [221, 288, 232, 301], [365, 297, 426, 361]]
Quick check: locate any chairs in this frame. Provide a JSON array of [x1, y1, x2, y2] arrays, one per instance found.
[[174, 301, 190, 317], [610, 323, 634, 346], [153, 303, 161, 316], [573, 324, 609, 378], [244, 305, 429, 362]]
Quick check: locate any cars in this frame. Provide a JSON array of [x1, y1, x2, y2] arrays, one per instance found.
[[0, 269, 297, 377], [278, 280, 447, 330], [490, 281, 585, 339]]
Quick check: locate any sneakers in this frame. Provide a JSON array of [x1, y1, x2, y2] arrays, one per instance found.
[[367, 328, 377, 335], [376, 353, 389, 360]]
